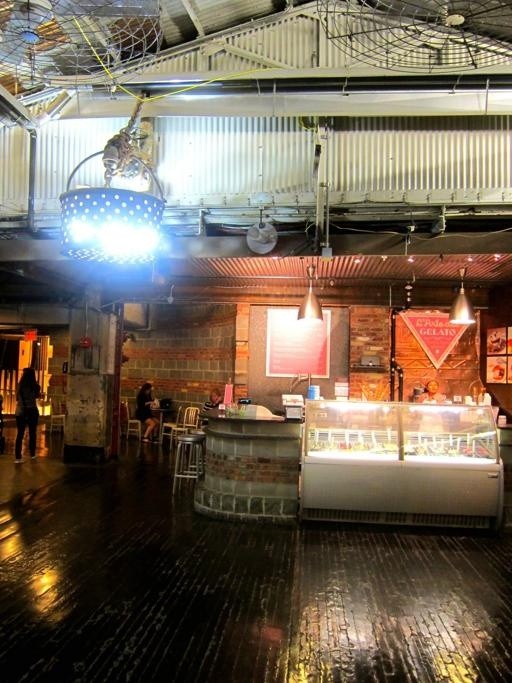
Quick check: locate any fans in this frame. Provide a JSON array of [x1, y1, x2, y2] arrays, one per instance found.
[[1, 0, 164, 92], [316, 0, 512, 75]]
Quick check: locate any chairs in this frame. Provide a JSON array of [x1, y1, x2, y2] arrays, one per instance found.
[[49, 396, 204, 452]]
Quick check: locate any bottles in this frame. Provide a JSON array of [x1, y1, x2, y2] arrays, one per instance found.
[[63, 359, 68, 372], [239, 398, 251, 415]]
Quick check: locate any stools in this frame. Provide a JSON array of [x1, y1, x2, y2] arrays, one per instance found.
[[172, 434, 204, 497]]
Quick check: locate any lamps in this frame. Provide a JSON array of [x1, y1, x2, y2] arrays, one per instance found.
[[446, 266, 479, 324], [298, 264, 325, 323], [56, 91, 172, 267]]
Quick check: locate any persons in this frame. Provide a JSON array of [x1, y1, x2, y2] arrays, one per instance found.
[[14, 367, 46, 463], [416, 380, 445, 402], [199, 388, 223, 425], [135, 382, 159, 442]]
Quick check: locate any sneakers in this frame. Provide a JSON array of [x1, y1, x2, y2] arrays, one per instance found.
[[13, 459, 24, 463], [142, 438, 152, 442]]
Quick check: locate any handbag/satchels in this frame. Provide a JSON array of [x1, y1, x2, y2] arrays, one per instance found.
[[150, 397, 159, 409], [224, 377, 233, 405], [22, 406, 38, 421]]
